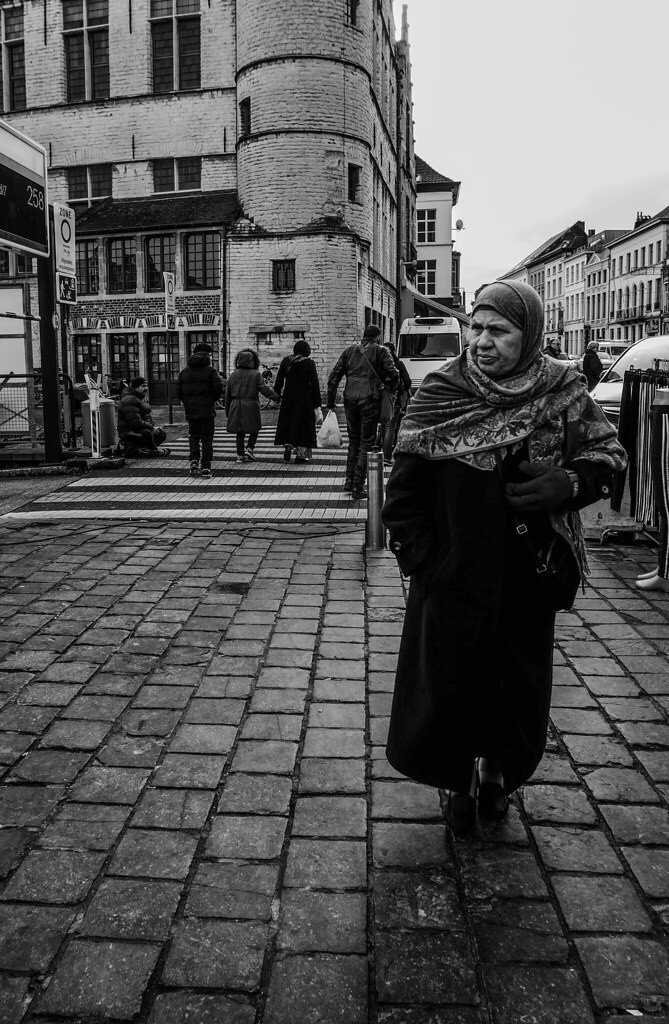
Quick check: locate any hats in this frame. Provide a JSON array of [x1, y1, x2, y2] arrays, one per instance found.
[[364, 325, 381, 337], [131, 377, 147, 389]]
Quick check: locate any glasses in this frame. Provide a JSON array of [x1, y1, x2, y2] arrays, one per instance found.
[[551, 343, 561, 346]]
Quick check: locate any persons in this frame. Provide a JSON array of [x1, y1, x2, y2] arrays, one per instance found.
[[273, 340, 322, 462], [374, 342, 412, 466], [116, 378, 171, 456], [178, 344, 223, 479], [583, 340, 602, 392], [542, 339, 562, 360], [381, 279, 628, 831], [218, 370, 226, 394], [225, 349, 280, 464], [327, 325, 400, 499]]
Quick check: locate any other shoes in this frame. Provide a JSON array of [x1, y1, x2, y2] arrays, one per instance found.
[[343, 482, 356, 491], [284, 446, 291, 461], [449, 773, 477, 830], [202, 468, 211, 476], [383, 459, 394, 466], [190, 462, 199, 476], [476, 756, 509, 818], [148, 447, 171, 457], [295, 457, 308, 463], [236, 455, 246, 463], [245, 450, 256, 461], [352, 490, 368, 499]]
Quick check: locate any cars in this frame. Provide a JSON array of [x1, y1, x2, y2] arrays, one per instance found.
[[559, 353, 575, 369], [589, 335, 669, 428], [578, 352, 615, 372], [569, 355, 582, 363]]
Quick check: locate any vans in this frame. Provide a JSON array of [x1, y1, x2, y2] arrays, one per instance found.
[[586, 339, 632, 361], [395, 316, 463, 388]]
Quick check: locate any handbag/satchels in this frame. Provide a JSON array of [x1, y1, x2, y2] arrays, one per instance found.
[[317, 409, 342, 447], [534, 534, 581, 614], [377, 388, 393, 421]]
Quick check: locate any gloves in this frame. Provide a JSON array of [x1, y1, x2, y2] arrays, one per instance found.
[[503, 461, 574, 515]]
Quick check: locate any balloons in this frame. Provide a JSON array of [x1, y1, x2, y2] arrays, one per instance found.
[[456, 219, 463, 232]]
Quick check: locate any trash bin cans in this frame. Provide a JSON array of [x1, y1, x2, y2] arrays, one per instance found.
[[81, 398, 117, 447]]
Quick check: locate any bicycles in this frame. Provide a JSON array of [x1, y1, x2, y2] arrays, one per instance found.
[[216, 379, 230, 409], [258, 363, 284, 410]]
